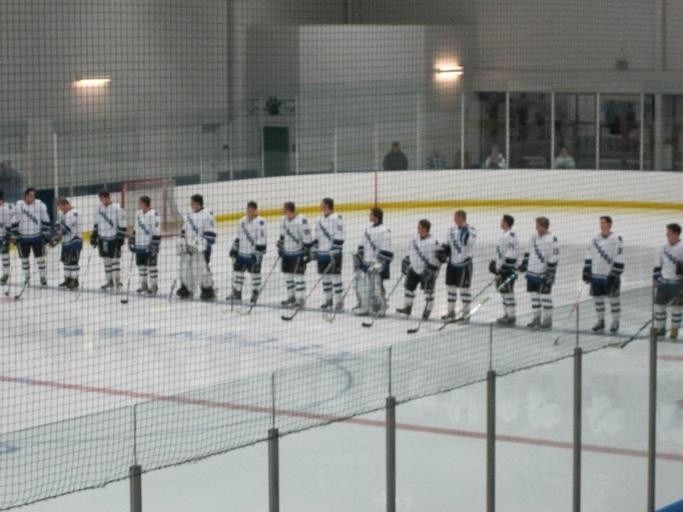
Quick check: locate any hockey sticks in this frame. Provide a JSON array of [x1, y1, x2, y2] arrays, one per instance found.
[[280, 262, 333, 320], [5, 276, 10, 296], [15, 278, 28, 299], [434, 272, 518, 334], [553, 284, 586, 346], [121, 251, 134, 304], [323, 273, 358, 323], [237, 258, 279, 316], [407, 287, 431, 333], [361, 273, 403, 327], [618, 318, 652, 349]]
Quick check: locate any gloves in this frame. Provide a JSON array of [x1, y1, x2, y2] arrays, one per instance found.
[[115, 227, 136, 252], [519, 252, 530, 273], [543, 262, 557, 287], [10, 230, 20, 244], [422, 263, 437, 284], [148, 233, 161, 258], [277, 234, 284, 259], [582, 258, 592, 284], [435, 242, 452, 262], [354, 246, 412, 276], [229, 237, 239, 258], [41, 230, 62, 247], [301, 245, 311, 263], [90, 225, 99, 247], [330, 238, 343, 259], [607, 260, 626, 285]]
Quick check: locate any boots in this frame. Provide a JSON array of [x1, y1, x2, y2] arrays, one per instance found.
[[526, 317, 552, 330], [281, 297, 303, 307], [593, 320, 619, 332], [1, 273, 9, 284], [321, 299, 345, 309], [496, 315, 516, 324], [653, 326, 678, 337], [178, 288, 217, 298], [441, 312, 471, 321], [423, 310, 431, 320], [225, 289, 257, 302], [135, 282, 158, 294], [58, 278, 80, 289], [39, 277, 48, 286], [397, 305, 412, 315], [101, 280, 122, 290]]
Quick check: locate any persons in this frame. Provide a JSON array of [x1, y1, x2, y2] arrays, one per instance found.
[[651, 223, 683, 341], [0, 161, 83, 290], [383, 141, 410, 170], [226, 202, 267, 303], [484, 144, 506, 171], [552, 148, 576, 171], [518, 217, 560, 331], [488, 215, 518, 326], [274, 196, 477, 324], [582, 216, 625, 331], [90, 189, 217, 305]]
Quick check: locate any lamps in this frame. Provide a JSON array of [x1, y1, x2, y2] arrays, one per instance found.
[[434, 66, 464, 75], [73, 71, 111, 83]]
